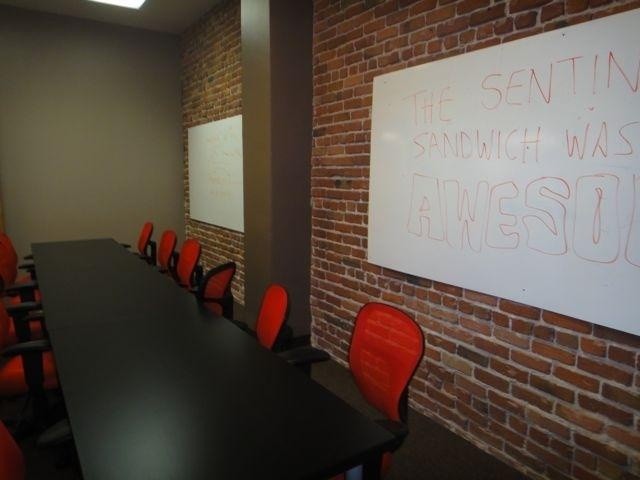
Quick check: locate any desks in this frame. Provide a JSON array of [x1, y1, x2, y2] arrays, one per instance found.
[[23, 235, 539, 475]]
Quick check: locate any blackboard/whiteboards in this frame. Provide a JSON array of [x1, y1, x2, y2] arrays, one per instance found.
[[187, 114, 245, 234], [366, 6, 640, 337]]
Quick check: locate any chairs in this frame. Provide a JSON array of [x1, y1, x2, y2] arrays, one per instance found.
[[159, 229, 177, 266], [0, 236, 60, 465], [343, 301, 427, 415], [200, 259, 235, 304], [138, 223, 153, 253], [175, 237, 201, 284], [251, 278, 290, 349]]
